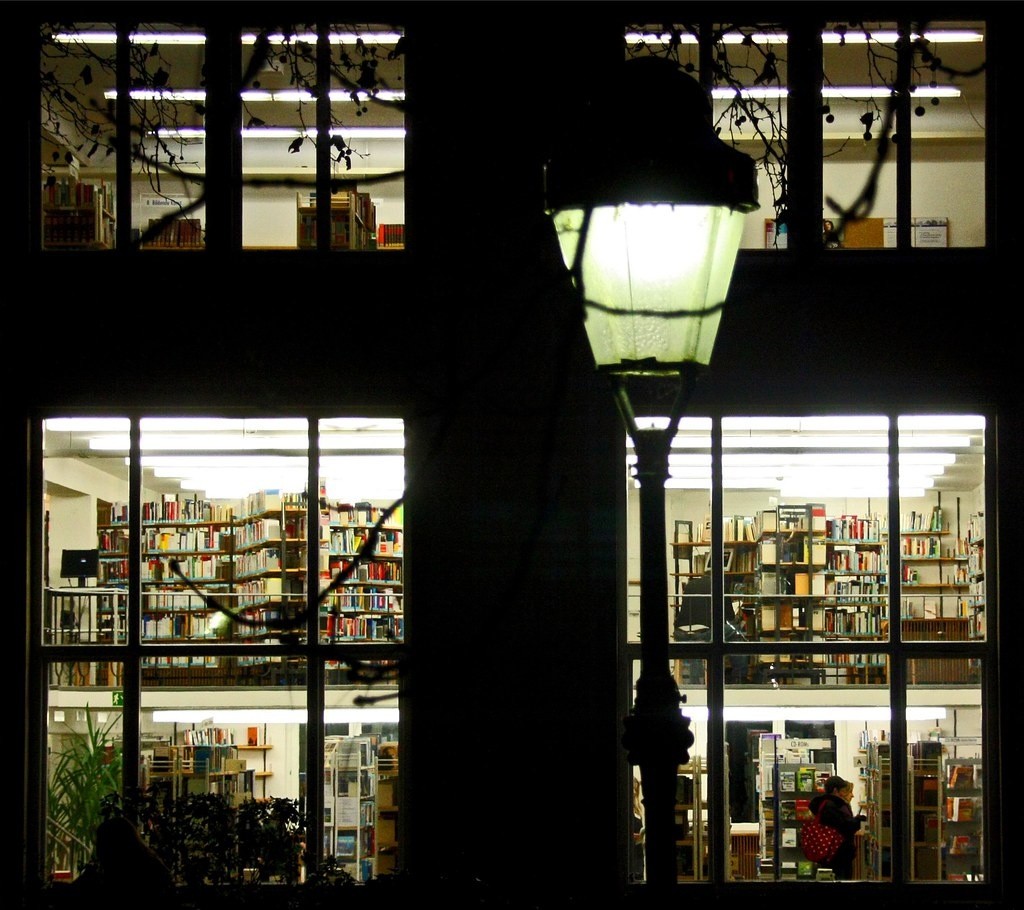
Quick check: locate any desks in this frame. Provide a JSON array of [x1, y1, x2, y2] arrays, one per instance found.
[[881, 617, 973, 685], [675, 830, 759, 883], [46, 587, 129, 688]]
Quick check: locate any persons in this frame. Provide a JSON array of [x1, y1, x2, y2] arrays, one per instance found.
[[674, 576, 750, 683], [843, 781, 855, 804], [632, 777, 644, 879], [824, 221, 834, 232], [809, 776, 861, 879]]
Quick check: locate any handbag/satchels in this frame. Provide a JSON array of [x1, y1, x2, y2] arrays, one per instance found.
[[800, 800, 846, 865]]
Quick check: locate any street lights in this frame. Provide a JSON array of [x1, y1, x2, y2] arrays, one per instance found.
[[538, 53, 760, 909]]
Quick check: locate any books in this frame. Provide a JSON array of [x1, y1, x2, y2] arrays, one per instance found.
[[748, 729, 982, 880], [153, 726, 239, 807], [300, 189, 404, 248], [691, 503, 986, 666], [40, 183, 112, 248], [97, 493, 403, 686], [146, 219, 201, 248], [674, 776, 693, 876], [635, 827, 646, 845], [322, 733, 398, 880]]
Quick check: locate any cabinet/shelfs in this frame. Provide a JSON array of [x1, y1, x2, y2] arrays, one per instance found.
[[296, 180, 377, 250], [675, 734, 985, 883], [323, 733, 399, 884], [96, 491, 403, 687], [670, 504, 985, 685], [41, 192, 115, 250], [113, 722, 273, 886]]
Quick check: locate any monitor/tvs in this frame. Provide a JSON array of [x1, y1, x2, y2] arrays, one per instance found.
[[59, 549, 99, 587]]
